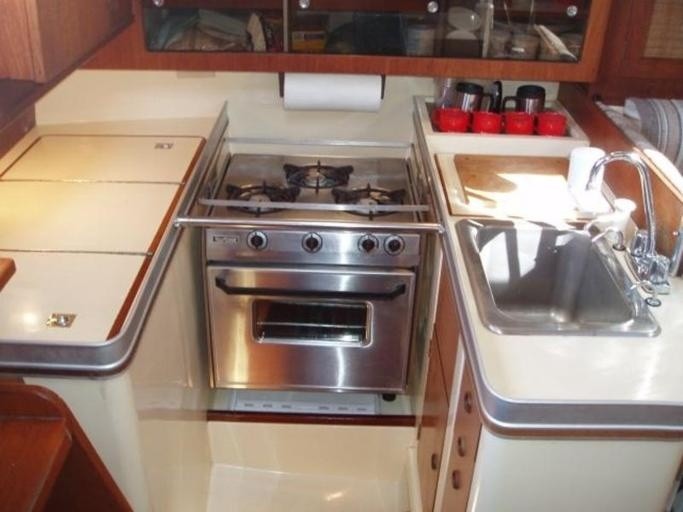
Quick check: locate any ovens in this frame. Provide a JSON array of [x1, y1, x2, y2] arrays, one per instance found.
[[201, 228, 421, 402]]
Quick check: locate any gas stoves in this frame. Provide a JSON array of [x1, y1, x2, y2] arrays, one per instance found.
[[220, 159, 407, 220]]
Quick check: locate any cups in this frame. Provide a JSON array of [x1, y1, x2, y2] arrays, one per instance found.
[[431, 107, 568, 135], [451, 82, 546, 112]]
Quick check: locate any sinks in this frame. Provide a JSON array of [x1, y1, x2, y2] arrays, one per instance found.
[[454, 217, 662, 340]]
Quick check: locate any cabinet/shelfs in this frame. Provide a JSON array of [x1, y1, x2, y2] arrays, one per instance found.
[[0, 1, 138, 86], [603, 1, 682, 83]]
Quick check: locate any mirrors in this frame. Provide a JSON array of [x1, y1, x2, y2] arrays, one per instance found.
[[76, 1, 612, 84]]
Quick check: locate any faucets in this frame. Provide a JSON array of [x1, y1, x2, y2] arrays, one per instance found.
[[585, 151, 657, 256], [590, 227, 625, 250]]
